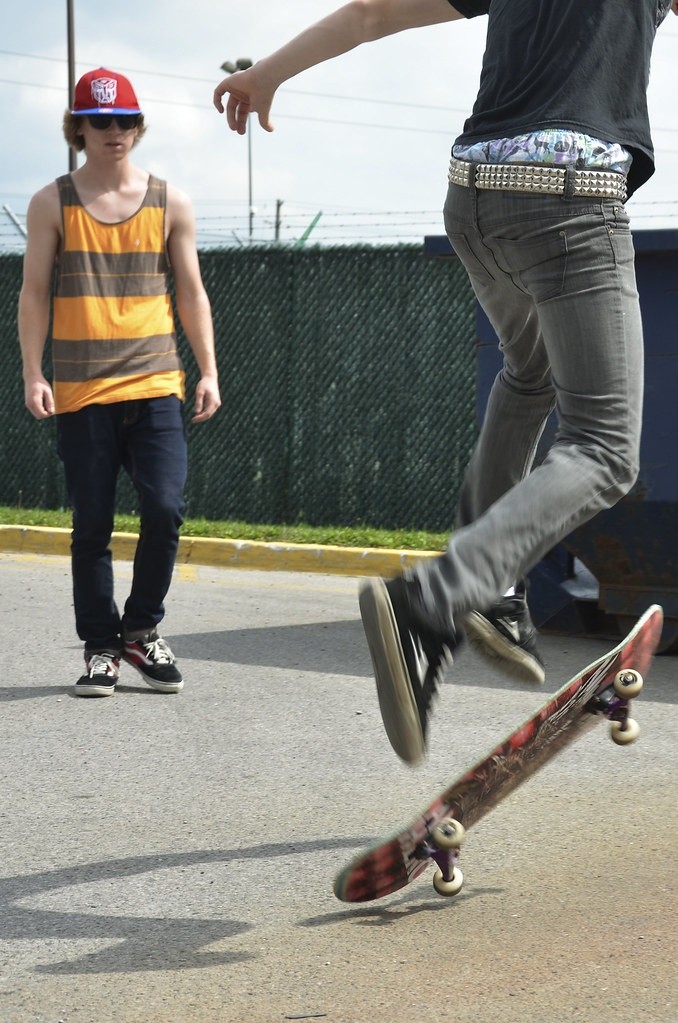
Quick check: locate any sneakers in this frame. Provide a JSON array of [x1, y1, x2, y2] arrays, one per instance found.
[[120, 631, 183, 691], [360, 580, 462, 763], [464, 580, 544, 687], [73, 650, 123, 697]]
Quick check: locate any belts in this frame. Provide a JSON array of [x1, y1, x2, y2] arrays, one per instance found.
[[449, 158, 628, 200]]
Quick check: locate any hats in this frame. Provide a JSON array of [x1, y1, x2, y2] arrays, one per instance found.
[[71, 66, 143, 116]]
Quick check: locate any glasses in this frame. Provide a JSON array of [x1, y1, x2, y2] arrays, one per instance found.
[[80, 115, 139, 131]]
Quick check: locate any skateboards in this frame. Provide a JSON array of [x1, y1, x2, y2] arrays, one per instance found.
[[333, 604, 663, 903]]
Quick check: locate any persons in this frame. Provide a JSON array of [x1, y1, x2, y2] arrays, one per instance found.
[[17, 68, 222, 695], [214, 0, 678, 764]]
